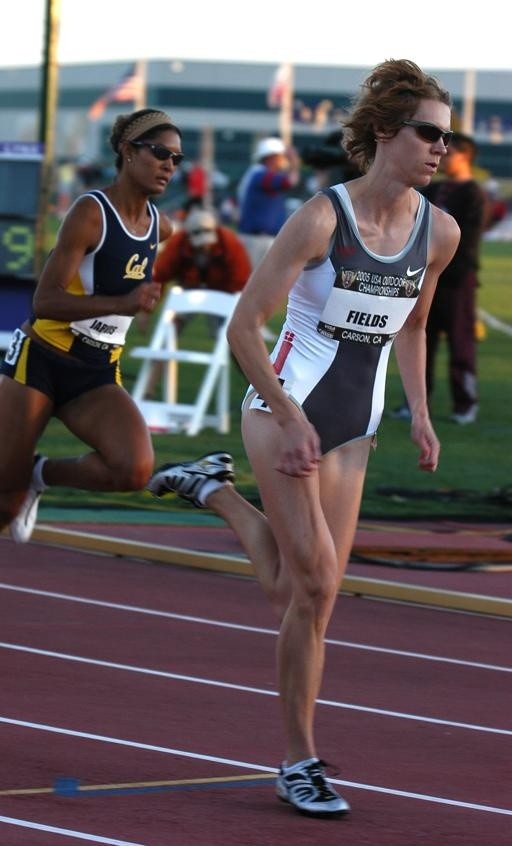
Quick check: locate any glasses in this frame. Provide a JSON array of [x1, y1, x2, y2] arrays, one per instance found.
[[404, 119, 453, 147], [119, 137, 185, 166]]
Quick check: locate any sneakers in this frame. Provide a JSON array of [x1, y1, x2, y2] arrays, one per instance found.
[[147, 451, 237, 510], [274, 756, 351, 818], [9, 450, 44, 545]]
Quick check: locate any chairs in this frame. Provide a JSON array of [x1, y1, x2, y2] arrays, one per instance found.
[[121, 283, 242, 441]]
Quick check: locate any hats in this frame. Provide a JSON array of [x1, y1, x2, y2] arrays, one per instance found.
[[184, 211, 218, 246], [250, 137, 286, 163]]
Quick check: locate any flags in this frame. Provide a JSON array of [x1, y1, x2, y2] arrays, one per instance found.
[[267, 62, 291, 107], [87, 60, 139, 120]]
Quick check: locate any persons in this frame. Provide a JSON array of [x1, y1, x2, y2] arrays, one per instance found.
[[0, 109, 185, 544], [146, 139, 327, 397], [394, 132, 490, 424], [146, 57, 462, 819]]
